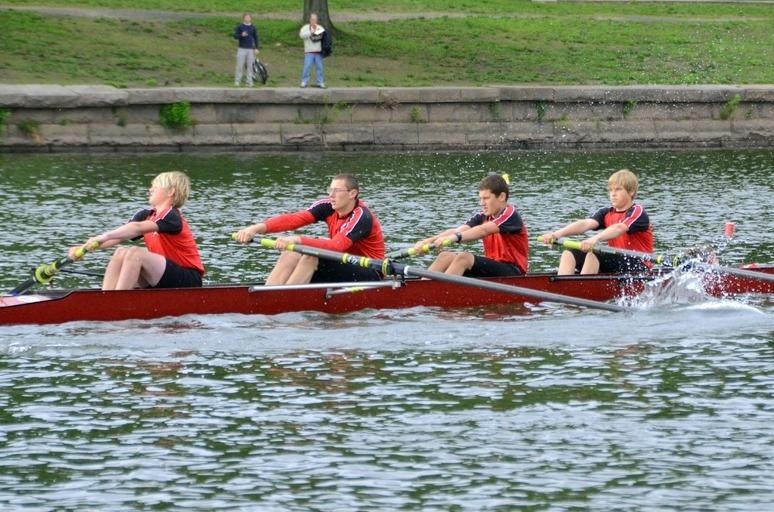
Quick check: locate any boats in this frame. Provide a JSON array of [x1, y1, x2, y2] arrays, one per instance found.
[[0, 262, 769, 327]]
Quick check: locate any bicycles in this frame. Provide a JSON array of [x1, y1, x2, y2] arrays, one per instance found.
[[231, 33, 270, 85]]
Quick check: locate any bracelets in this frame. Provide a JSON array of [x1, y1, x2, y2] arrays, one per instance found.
[[455, 232, 461, 243]]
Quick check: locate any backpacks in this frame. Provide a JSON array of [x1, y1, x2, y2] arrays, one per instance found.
[[321, 30, 330, 57]]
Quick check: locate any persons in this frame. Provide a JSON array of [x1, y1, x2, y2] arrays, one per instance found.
[[68, 171, 204, 291], [299, 13, 326, 88], [231, 11, 260, 87], [542, 169, 654, 276], [235, 174, 386, 287], [414, 174, 528, 281]]
